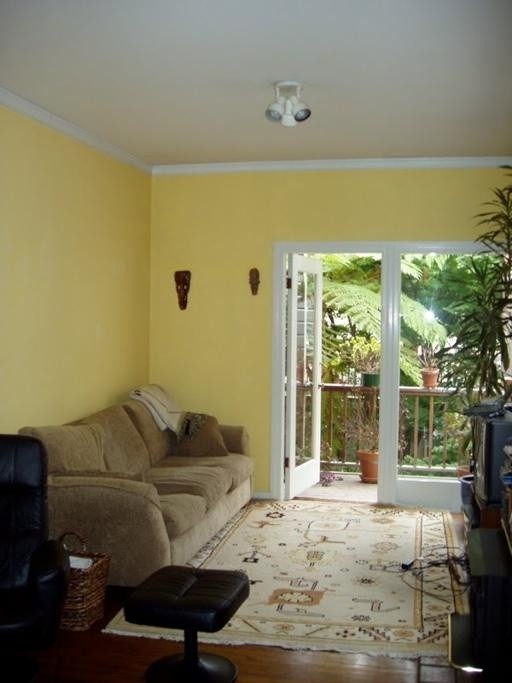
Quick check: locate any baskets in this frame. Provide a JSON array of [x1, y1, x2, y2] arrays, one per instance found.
[[59, 531, 109, 633]]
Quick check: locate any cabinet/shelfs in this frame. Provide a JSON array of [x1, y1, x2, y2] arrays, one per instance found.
[[449, 515, 511, 683]]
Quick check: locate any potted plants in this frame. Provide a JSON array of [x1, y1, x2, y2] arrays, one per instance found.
[[355, 344, 386, 386], [435, 179, 510, 509], [356, 388, 409, 485], [416, 341, 444, 391]]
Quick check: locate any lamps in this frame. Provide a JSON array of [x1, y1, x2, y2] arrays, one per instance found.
[[262, 80, 311, 128]]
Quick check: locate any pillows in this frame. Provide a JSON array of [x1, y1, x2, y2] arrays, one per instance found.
[[170, 408, 229, 458]]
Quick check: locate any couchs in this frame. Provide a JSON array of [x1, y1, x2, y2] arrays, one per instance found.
[[18, 398, 257, 601]]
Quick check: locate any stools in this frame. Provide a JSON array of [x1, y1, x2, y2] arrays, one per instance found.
[[125, 564, 251, 683]]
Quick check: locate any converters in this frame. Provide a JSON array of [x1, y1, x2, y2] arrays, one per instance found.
[[401, 559, 414, 570]]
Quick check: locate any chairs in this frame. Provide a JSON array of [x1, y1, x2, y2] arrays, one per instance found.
[[0, 433, 71, 682]]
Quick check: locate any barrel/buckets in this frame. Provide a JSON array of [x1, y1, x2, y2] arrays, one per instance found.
[[460, 474, 474, 504]]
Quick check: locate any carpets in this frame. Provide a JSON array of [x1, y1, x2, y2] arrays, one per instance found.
[[99, 492, 475, 662]]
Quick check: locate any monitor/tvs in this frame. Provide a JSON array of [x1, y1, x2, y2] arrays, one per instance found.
[[473, 409, 512, 506]]
